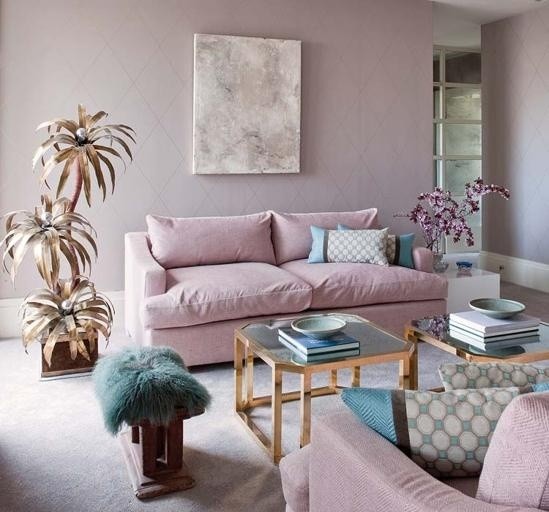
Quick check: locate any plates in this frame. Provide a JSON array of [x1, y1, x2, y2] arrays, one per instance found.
[[290, 315, 346, 339], [469, 297, 526, 318]]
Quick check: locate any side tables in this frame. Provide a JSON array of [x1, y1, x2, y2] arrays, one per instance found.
[[434, 266, 503, 312]]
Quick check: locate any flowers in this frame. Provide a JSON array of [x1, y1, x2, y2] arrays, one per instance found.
[[400, 177, 511, 250]]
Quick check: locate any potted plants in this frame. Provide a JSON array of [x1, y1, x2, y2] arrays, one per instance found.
[[1, 105, 136, 381]]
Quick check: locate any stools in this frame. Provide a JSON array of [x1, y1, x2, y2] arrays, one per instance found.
[[91, 347, 211, 500]]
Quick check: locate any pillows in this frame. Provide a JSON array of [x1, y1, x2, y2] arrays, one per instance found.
[[336, 223, 419, 268], [309, 225, 390, 265], [437, 360, 549, 394], [269, 208, 380, 267], [340, 386, 523, 479], [144, 210, 277, 269], [474, 391, 548, 512]]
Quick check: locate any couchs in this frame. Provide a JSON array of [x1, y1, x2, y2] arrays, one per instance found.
[[278, 411, 549, 512], [122, 208, 451, 368]]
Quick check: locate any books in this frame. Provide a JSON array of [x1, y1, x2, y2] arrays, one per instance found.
[[277, 328, 359, 355], [449, 308, 541, 344]]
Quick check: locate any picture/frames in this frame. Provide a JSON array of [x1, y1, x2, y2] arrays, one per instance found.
[[193, 34, 302, 176]]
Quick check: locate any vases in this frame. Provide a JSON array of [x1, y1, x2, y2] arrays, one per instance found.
[[431, 255, 448, 273]]
